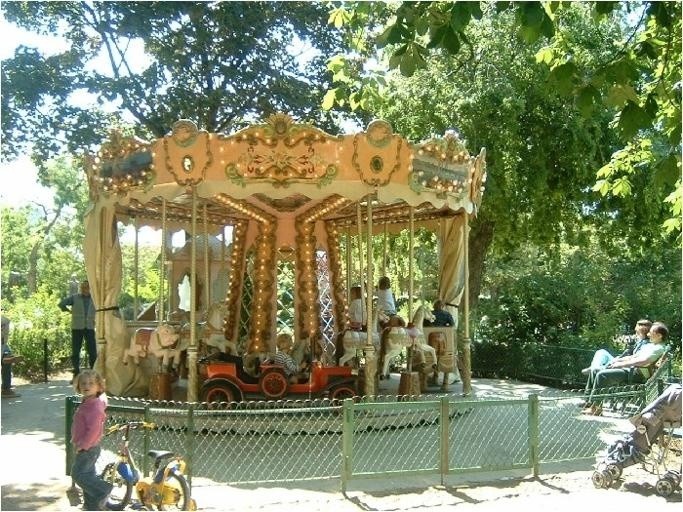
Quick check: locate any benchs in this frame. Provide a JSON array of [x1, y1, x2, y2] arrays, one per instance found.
[[583, 341, 673, 415]]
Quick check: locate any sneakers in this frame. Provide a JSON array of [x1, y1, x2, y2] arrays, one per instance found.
[[2, 390, 20, 397], [2, 354, 23, 365], [99, 484, 112, 508], [581, 367, 603, 375], [579, 400, 603, 416]]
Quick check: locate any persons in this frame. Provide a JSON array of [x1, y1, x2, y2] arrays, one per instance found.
[[424, 301, 454, 328], [346, 286, 363, 329], [181, 312, 190, 326], [375, 276, 397, 326], [56, 279, 100, 384], [580, 322, 670, 418], [276, 334, 326, 383], [576, 319, 653, 409], [1, 308, 26, 398], [69, 368, 114, 510]]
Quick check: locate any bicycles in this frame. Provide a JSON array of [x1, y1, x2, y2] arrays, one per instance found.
[[98, 419, 195, 510]]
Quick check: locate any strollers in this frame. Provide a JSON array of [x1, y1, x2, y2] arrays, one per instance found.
[[588, 377, 682, 496]]
